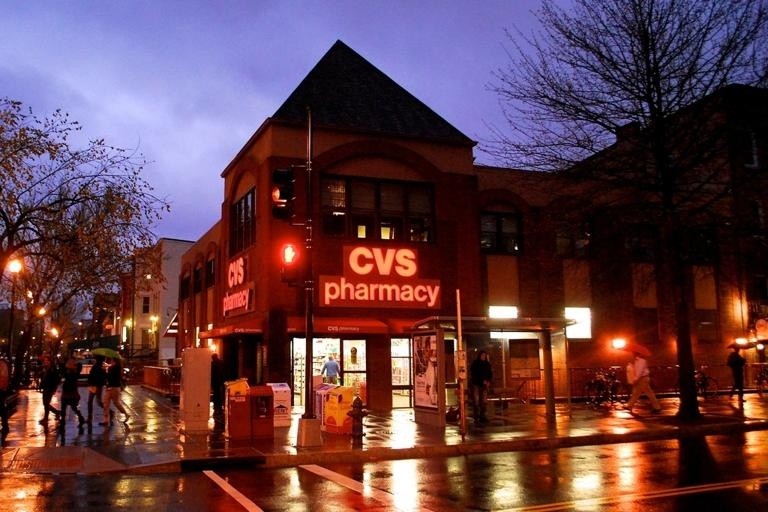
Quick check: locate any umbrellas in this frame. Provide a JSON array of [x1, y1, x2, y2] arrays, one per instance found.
[[723, 337, 757, 351], [82, 347, 123, 359], [616, 341, 652, 358]]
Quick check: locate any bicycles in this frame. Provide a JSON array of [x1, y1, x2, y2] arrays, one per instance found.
[[672, 365, 718, 399], [584, 366, 630, 406]]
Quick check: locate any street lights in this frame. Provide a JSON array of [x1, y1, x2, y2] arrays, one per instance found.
[[6, 259, 60, 357]]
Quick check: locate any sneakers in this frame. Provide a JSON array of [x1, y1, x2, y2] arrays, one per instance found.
[[39, 410, 130, 429]]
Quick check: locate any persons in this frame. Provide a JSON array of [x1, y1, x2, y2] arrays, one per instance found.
[[208, 352, 224, 409], [726, 346, 747, 403], [623, 351, 661, 414], [54, 358, 85, 429], [83, 356, 114, 425], [98, 359, 132, 426], [321, 355, 340, 385], [36, 357, 59, 423], [467, 349, 494, 424]]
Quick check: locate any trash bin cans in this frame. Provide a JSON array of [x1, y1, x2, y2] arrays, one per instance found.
[[250, 386, 274, 440]]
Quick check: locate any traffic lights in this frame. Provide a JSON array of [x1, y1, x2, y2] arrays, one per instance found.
[[272, 170, 296, 219], [272, 238, 306, 272]]
